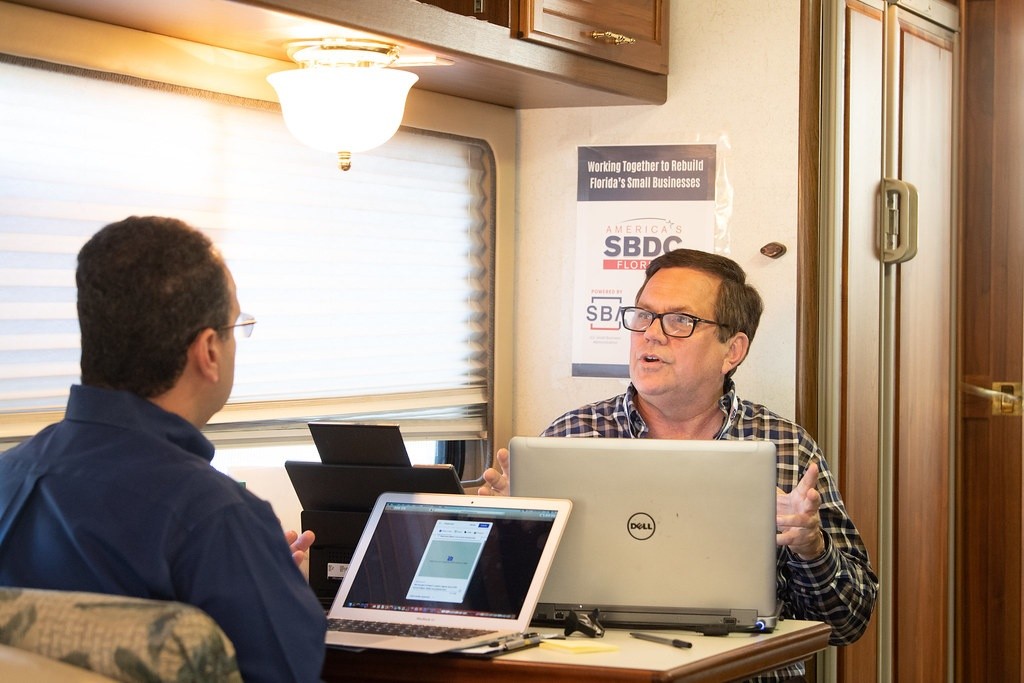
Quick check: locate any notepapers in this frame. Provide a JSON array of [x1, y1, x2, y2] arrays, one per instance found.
[[539, 638, 617, 653]]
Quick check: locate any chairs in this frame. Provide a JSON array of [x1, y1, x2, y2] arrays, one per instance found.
[[0, 586, 245, 682]]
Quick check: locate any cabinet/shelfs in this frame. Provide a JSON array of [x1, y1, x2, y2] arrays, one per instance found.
[[2, 0, 670, 112]]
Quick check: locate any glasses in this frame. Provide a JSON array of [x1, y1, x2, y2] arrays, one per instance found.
[[216, 313, 256, 338], [622, 306, 732, 339]]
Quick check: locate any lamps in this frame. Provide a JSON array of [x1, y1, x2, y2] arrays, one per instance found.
[[267, 38, 420, 171]]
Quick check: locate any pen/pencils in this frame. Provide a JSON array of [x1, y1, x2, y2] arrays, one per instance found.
[[489, 632, 539, 647], [629, 632, 693, 649]]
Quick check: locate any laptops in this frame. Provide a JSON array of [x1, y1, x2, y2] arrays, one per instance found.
[[509, 437, 785, 633], [325, 490, 573, 654]]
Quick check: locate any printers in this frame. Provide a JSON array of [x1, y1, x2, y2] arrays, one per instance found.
[[284, 422, 465, 609]]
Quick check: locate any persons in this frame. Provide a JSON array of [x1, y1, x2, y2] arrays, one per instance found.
[[478, 248, 879, 683], [0, 215, 327, 683]]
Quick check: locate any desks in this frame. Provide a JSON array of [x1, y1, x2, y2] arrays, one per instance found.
[[321, 620, 832, 683]]
